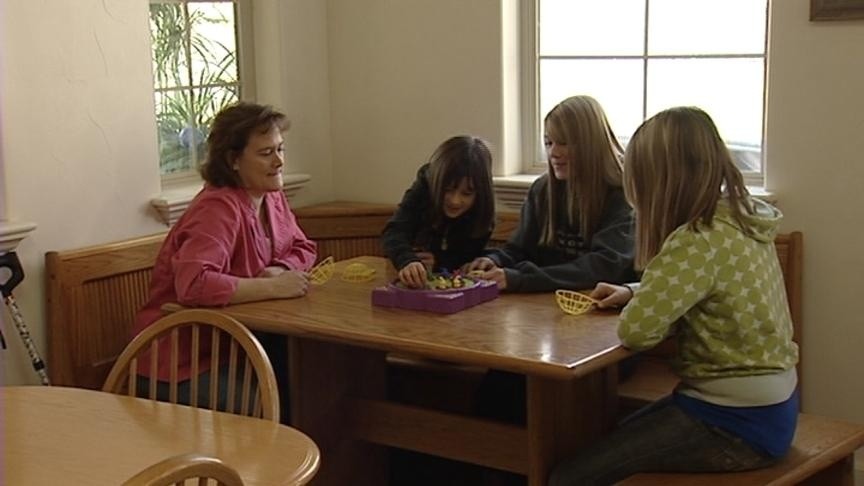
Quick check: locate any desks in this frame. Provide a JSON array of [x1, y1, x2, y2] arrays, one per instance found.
[[161, 255, 682, 485], [0, 385, 322, 485]]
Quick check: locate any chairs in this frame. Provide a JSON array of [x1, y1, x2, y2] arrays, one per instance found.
[[120, 453, 244, 485], [101, 308, 281, 424]]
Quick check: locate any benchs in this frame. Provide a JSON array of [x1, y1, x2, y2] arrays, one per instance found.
[[45, 200, 803, 414]]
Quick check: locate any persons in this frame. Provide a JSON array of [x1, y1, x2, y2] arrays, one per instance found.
[[133, 101, 317, 420], [545, 107, 800, 485], [455, 95, 643, 423], [381, 134, 497, 289]]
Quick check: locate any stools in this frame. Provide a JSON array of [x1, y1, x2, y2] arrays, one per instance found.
[[613, 413, 864, 486]]
